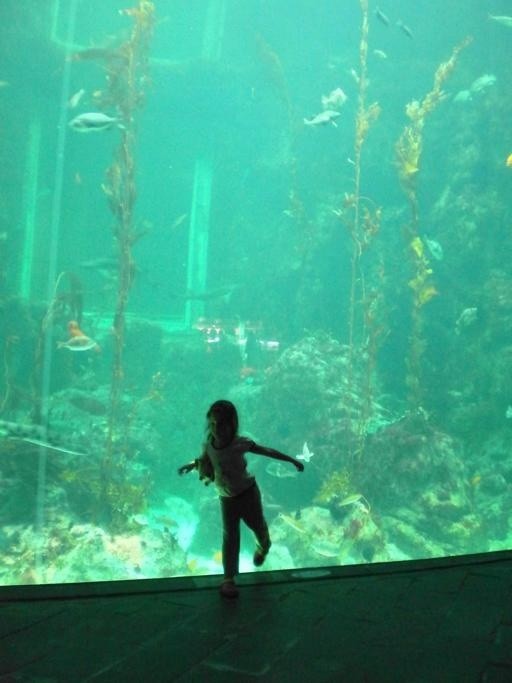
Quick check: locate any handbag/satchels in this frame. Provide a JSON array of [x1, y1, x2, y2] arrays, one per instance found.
[[195, 458, 215, 487]]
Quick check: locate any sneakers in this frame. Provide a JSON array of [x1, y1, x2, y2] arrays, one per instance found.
[[253, 542, 270, 567], [219, 579, 238, 604]]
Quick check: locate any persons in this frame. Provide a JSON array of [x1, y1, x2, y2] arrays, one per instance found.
[[178, 400, 304, 598]]
